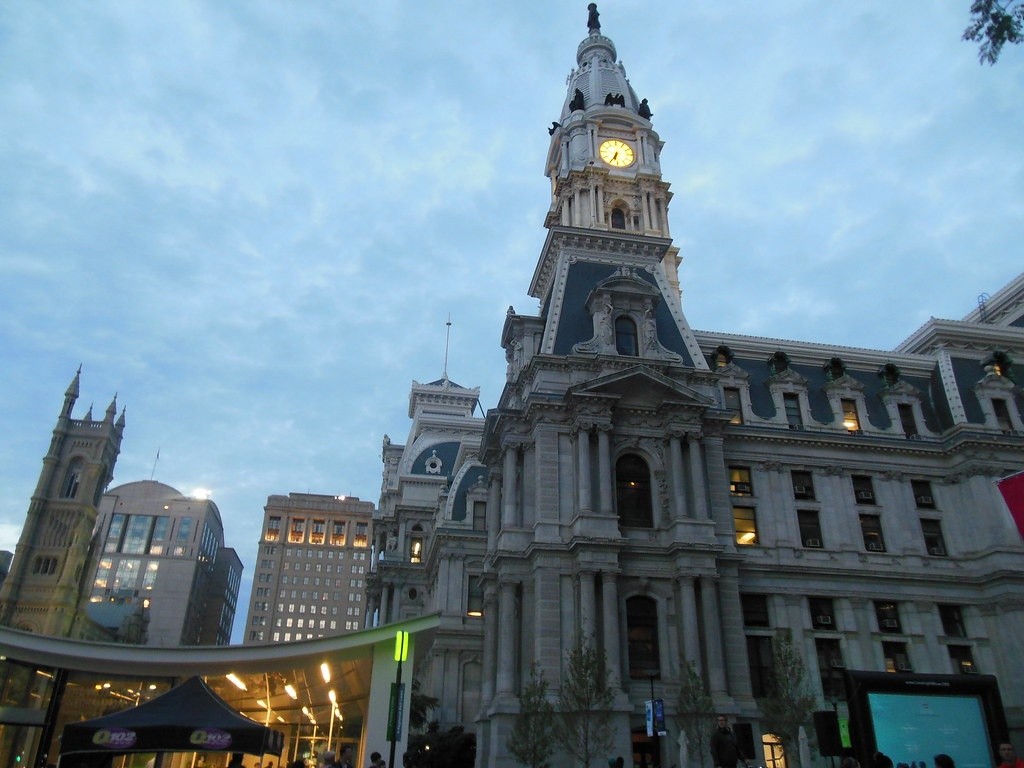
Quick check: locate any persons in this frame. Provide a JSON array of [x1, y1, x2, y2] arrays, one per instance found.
[[710, 713, 750, 768], [840, 751, 956, 768], [998, 742, 1024, 768], [294, 745, 386, 768]]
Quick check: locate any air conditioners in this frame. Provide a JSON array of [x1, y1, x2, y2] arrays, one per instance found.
[[882, 618, 899, 628], [920, 494, 933, 505], [795, 485, 806, 493], [852, 430, 864, 435], [832, 659, 846, 668], [794, 424, 805, 431], [859, 491, 873, 499], [930, 547, 946, 557], [869, 541, 884, 551], [736, 484, 748, 492], [910, 434, 924, 441], [817, 615, 833, 626], [900, 663, 912, 671], [807, 538, 821, 547], [964, 665, 979, 673]]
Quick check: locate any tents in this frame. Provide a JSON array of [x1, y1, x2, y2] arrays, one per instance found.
[[57, 674, 285, 768]]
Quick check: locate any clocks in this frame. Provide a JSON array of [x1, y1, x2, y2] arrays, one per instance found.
[[599, 139, 634, 167]]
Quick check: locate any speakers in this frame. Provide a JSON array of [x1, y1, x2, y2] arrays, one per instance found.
[[732, 723, 755, 759], [813, 711, 844, 757]]
